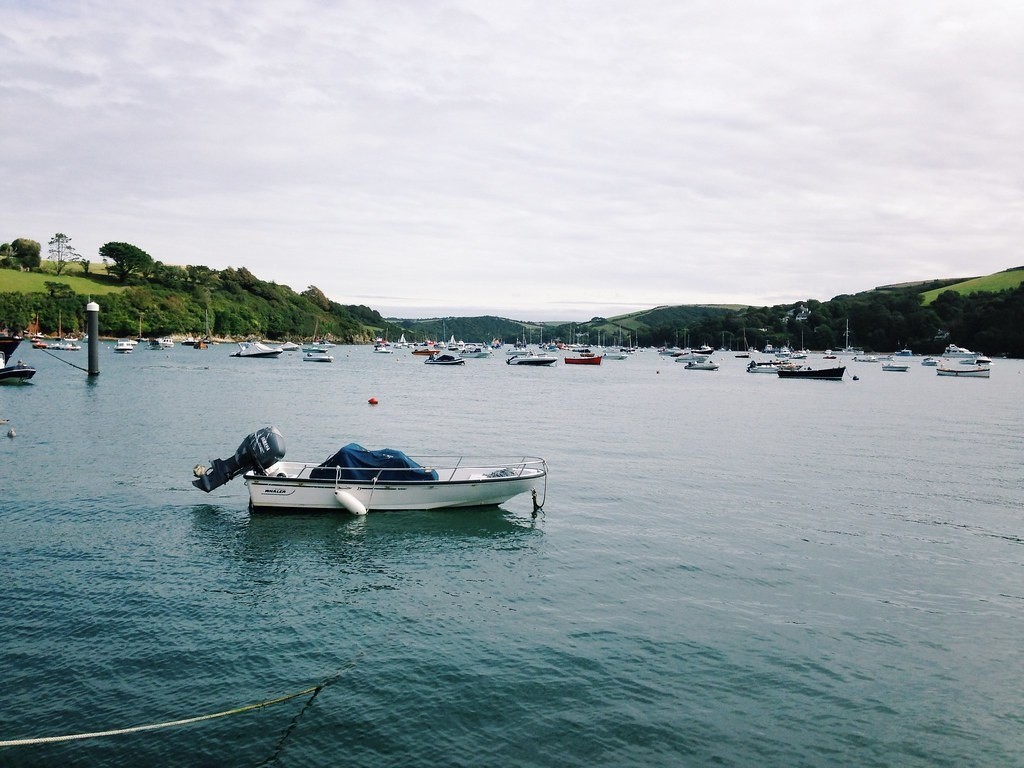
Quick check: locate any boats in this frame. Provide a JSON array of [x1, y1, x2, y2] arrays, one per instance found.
[[776, 366, 847, 381], [505, 352, 558, 366], [942, 344, 983, 357], [960, 357, 992, 365], [423, 353, 465, 366], [936, 364, 990, 378], [852, 355, 878, 362], [0, 333, 25, 366], [457, 347, 492, 358], [894, 350, 914, 356], [274, 341, 300, 351], [921, 360, 939, 366], [603, 354, 628, 360], [374, 348, 393, 354], [159, 338, 175, 348], [683, 361, 721, 371], [229, 341, 283, 358], [0, 351, 36, 383], [181, 337, 211, 346], [191, 428, 549, 516], [114, 338, 134, 354], [32, 341, 82, 351], [579, 352, 596, 357], [878, 356, 894, 361], [144, 345, 163, 350], [562, 353, 602, 365], [312, 339, 337, 349], [881, 364, 910, 372], [746, 359, 802, 373], [303, 353, 334, 362], [411, 349, 442, 355], [302, 347, 329, 353]]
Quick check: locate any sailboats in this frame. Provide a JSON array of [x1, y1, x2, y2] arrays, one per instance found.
[[657, 329, 812, 359], [374, 318, 639, 353], [823, 318, 865, 356]]
